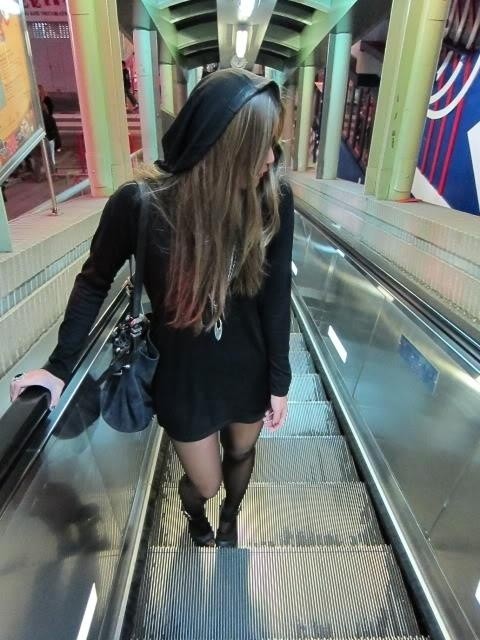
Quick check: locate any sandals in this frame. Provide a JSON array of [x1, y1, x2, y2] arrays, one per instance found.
[[188, 516, 213, 546], [216, 497, 238, 547]]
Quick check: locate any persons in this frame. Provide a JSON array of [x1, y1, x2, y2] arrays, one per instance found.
[[122, 60, 138, 110], [37, 84, 62, 152], [10, 66, 295, 547]]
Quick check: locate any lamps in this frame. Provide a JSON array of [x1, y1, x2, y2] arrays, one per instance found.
[[236, 24, 249, 58]]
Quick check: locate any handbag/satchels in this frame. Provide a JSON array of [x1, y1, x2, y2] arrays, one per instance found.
[[100, 313, 160, 432]]
